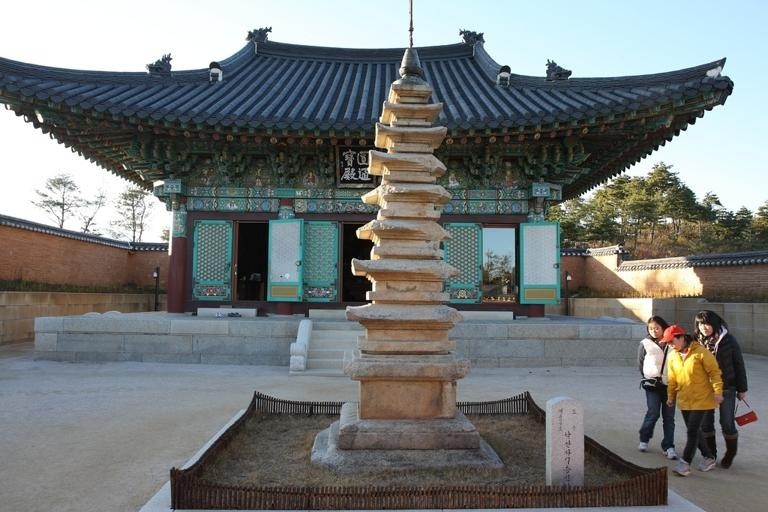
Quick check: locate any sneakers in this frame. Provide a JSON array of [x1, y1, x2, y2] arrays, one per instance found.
[[696, 457, 717, 473], [663, 446, 679, 460], [671, 457, 693, 477], [638, 442, 649, 452], [215, 312, 242, 318]]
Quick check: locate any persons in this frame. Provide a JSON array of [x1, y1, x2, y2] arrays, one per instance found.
[[694, 310, 748, 468], [635, 316, 677, 460], [658, 324, 723, 477]]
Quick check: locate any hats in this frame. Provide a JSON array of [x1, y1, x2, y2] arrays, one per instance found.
[[658, 324, 688, 345]]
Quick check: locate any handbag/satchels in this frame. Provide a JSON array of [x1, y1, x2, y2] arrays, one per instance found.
[[638, 375, 663, 393], [734, 398, 758, 427]]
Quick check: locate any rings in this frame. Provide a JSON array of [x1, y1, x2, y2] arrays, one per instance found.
[[740, 396, 742, 398]]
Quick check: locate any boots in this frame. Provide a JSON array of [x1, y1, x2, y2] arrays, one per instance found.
[[703, 430, 718, 459], [721, 432, 740, 469]]
